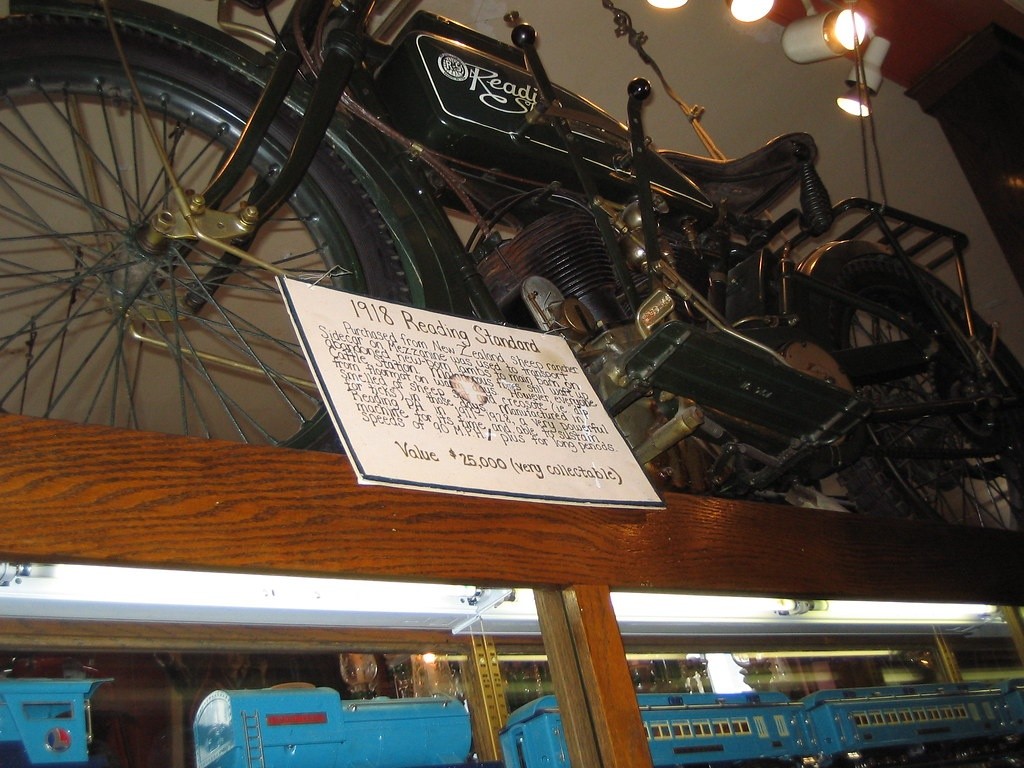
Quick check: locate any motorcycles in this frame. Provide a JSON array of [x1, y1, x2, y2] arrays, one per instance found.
[[1, 1, 1023, 537]]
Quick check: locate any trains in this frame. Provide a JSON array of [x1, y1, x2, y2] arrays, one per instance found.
[[1, 677, 115, 768], [192, 680, 1024, 768]]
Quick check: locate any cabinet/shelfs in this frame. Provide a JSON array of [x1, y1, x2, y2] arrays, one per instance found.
[[0, 408, 1024, 768]]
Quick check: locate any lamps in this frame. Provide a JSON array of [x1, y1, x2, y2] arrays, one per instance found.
[[835, 29, 895, 120], [780, 0, 868, 67]]
